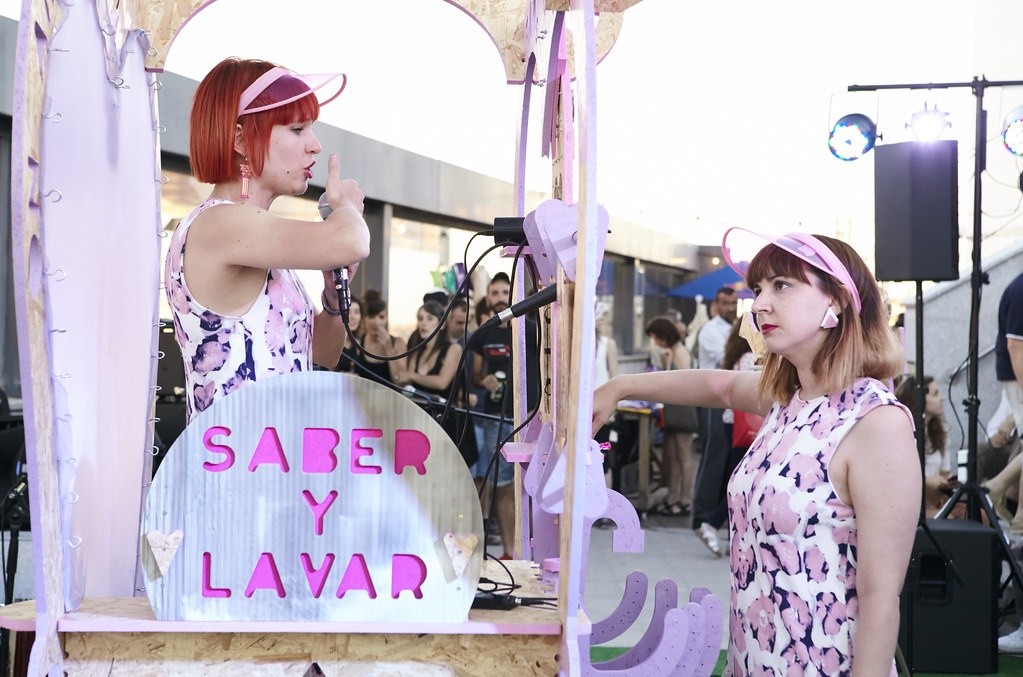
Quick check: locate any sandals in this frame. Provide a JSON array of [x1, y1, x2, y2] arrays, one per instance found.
[[660, 499, 691, 516], [646, 499, 669, 514]]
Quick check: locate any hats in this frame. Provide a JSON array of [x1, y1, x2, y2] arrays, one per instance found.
[[720, 226, 862, 317], [236, 65, 348, 118], [490, 268, 513, 285]]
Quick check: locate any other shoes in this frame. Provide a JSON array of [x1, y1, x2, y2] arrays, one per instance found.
[[599, 518, 609, 529], [695, 523, 721, 557], [997, 623, 1023, 659]]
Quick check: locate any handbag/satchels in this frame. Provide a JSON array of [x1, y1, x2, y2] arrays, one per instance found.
[[731, 404, 764, 447]]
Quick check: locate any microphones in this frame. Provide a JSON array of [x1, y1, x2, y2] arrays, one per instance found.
[[317, 192, 350, 322], [494, 594, 542, 606], [475, 284, 558, 336]]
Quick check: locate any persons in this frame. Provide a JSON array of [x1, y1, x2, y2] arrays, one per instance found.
[[896, 272, 1023, 536], [342, 274, 513, 557], [592, 328, 614, 530], [166, 58, 372, 424], [647, 289, 768, 559], [595, 236, 922, 676]]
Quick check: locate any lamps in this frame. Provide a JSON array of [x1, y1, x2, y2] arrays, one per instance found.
[[827, 88, 883, 159], [1001, 108, 1023, 158], [903, 86, 949, 141]]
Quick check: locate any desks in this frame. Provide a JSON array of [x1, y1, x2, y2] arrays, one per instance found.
[[616, 405, 671, 519]]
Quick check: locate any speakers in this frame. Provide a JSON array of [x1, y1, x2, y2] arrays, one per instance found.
[[900, 518, 1003, 677], [874, 140, 960, 282]]
